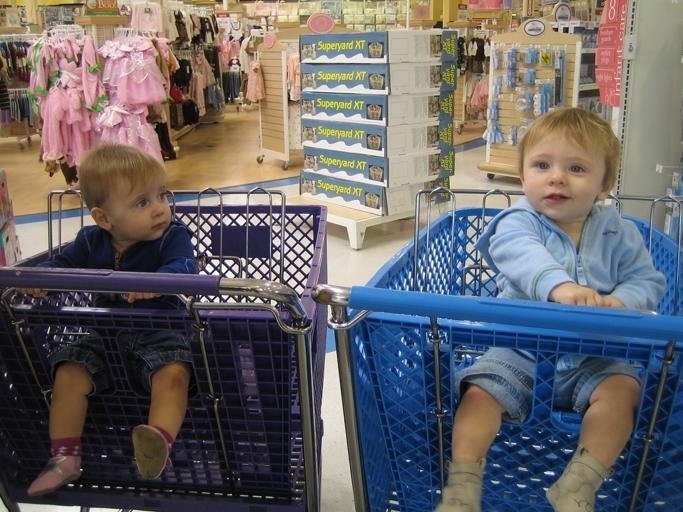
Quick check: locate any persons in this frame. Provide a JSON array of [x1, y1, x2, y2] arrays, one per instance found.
[[430, 103, 669, 512], [14, 142, 200, 497]]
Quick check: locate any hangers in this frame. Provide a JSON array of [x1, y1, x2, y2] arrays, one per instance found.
[[163, 0, 215, 21], [109, 26, 157, 53], [169, 42, 206, 60], [188, 67, 203, 80], [0, 33, 34, 48], [7, 87, 32, 102], [137, 0, 158, 9], [38, 25, 89, 52], [205, 77, 220, 89]]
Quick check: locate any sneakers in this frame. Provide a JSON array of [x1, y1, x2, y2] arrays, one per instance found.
[[28, 454, 85, 497], [133, 424, 173, 484]]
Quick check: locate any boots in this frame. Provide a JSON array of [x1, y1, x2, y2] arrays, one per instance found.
[[545, 443, 612, 512], [432, 460, 485, 512]]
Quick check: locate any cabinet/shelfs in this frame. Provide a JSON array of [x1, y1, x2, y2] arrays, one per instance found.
[[253, 19, 614, 250]]
